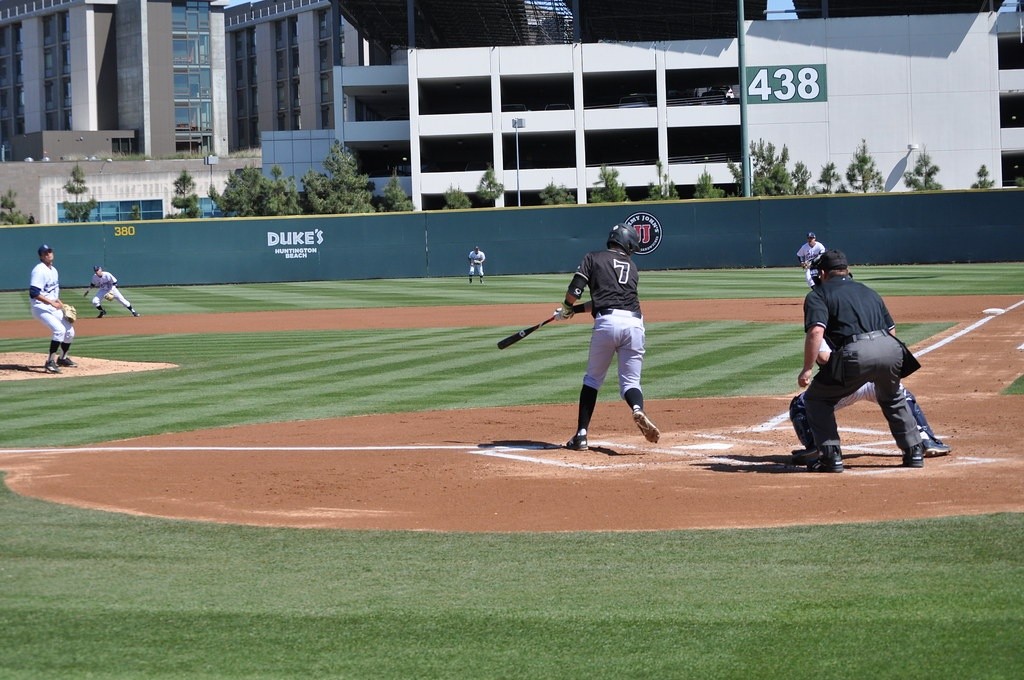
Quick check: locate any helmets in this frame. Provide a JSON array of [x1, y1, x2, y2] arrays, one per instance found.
[[607, 222, 640, 256]]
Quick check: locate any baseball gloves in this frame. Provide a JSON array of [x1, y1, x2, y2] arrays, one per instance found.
[[61, 304, 77, 323], [801, 262, 808, 269], [474, 260, 481, 265], [104, 292, 115, 301]]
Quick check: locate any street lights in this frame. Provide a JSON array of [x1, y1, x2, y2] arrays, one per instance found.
[[204, 155, 219, 217], [512, 118, 526, 205]]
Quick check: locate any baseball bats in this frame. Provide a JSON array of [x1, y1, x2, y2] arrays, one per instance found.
[[496, 316, 556, 350]]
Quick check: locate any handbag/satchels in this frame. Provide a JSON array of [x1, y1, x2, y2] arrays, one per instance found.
[[885, 329, 921, 379]]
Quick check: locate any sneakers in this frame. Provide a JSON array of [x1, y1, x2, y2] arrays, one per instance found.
[[45, 360, 63, 374], [922, 437, 951, 457], [807, 445, 844, 473], [791, 447, 819, 464], [55, 356, 77, 367], [566, 434, 589, 451], [902, 442, 926, 467], [632, 408, 660, 443]]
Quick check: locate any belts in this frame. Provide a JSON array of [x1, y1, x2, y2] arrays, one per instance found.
[[594, 308, 641, 319], [835, 329, 888, 351]]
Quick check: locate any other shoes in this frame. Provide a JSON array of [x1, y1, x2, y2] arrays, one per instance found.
[[98, 309, 106, 318], [130, 311, 140, 317]]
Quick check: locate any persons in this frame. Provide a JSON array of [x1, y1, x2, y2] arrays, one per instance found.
[[788, 248, 953, 474], [553, 223, 661, 451], [29, 244, 78, 374], [83, 265, 141, 319], [468, 246, 486, 285], [796, 232, 826, 291]]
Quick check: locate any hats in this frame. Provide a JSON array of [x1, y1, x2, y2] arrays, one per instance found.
[[817, 248, 847, 270], [38, 244, 52, 256], [94, 264, 102, 270], [807, 232, 815, 238], [474, 246, 479, 249]]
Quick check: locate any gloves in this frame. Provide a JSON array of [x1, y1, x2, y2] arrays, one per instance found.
[[553, 299, 575, 321]]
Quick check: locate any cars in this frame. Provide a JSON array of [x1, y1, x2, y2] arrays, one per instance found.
[[618, 95, 649, 108], [545, 103, 571, 110], [699, 91, 731, 104], [502, 104, 526, 112], [709, 86, 734, 98]]
